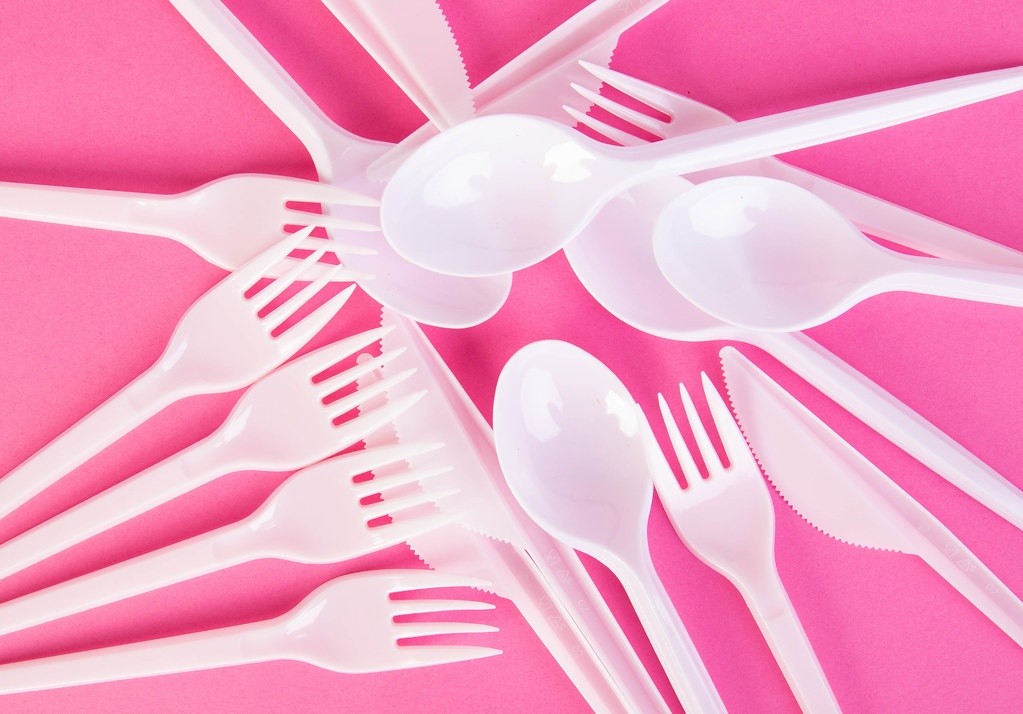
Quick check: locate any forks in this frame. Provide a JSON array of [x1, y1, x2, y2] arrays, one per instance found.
[[0, 173, 381, 282], [0, 569, 505, 695], [0, 443, 462, 636], [0, 325, 428, 580], [0, 225, 357, 520], [635, 370, 843, 714], [563, 59, 1023, 279]]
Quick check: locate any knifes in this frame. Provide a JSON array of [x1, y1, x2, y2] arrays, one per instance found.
[[319, 0, 478, 133], [719, 345, 1023, 647], [368, 0, 669, 191]]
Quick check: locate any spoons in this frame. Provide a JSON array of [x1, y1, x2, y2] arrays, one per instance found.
[[379, 66, 1022, 279], [492, 339, 728, 713], [166, 0, 512, 330], [560, 174, 1023, 530], [652, 175, 1023, 333]]
